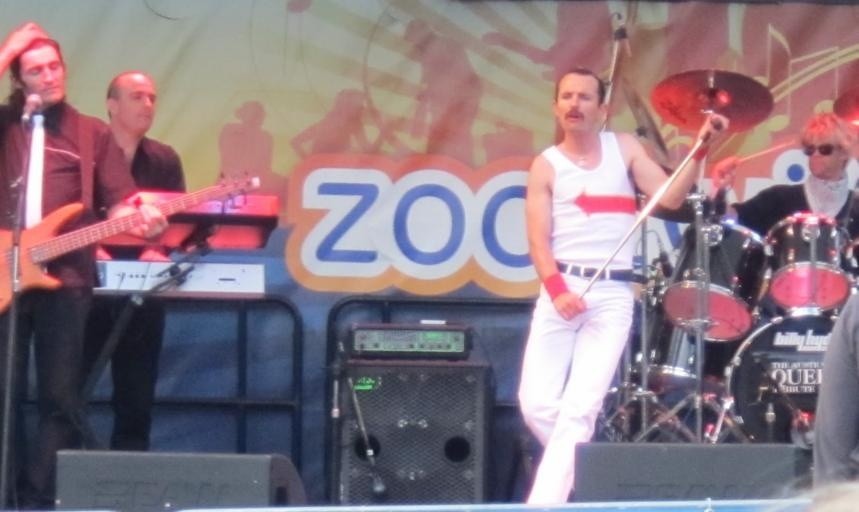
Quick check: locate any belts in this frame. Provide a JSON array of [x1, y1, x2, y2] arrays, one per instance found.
[[551, 257, 650, 287]]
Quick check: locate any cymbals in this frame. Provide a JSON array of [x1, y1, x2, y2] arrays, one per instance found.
[[619, 78, 668, 155], [651, 70, 772, 131], [628, 164, 710, 222]]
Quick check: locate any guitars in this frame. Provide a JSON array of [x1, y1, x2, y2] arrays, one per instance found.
[[0, 171, 253, 311]]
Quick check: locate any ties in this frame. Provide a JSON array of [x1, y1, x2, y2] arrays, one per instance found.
[[23, 111, 48, 227]]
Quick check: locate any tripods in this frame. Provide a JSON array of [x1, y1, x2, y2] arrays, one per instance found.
[[605, 340, 692, 444], [631, 190, 752, 445], [593, 192, 696, 444]]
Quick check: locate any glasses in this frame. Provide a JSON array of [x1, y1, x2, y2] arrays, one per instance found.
[[803, 142, 835, 157]]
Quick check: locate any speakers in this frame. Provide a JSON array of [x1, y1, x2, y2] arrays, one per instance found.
[[56, 450, 309, 510], [336, 357, 498, 505], [491, 433, 522, 503], [574, 443, 808, 503]]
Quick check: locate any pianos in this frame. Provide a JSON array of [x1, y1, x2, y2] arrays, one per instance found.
[[92, 256, 267, 306]]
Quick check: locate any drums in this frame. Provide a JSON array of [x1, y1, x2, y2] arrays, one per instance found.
[[664, 219, 770, 340], [629, 364, 729, 444], [767, 211, 852, 310], [729, 313, 841, 445]]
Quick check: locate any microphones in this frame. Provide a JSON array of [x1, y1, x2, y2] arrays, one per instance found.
[[21, 94, 44, 120], [789, 406, 814, 446], [660, 250, 673, 278], [710, 117, 723, 130], [617, 13, 633, 58]]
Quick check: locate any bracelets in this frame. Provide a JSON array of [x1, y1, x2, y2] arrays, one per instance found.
[[538, 271, 565, 306], [689, 143, 710, 163]]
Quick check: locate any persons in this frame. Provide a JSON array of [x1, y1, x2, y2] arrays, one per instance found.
[[700, 110, 858, 446], [0, 21, 170, 511], [75, 67, 189, 450], [515, 64, 734, 503]]
